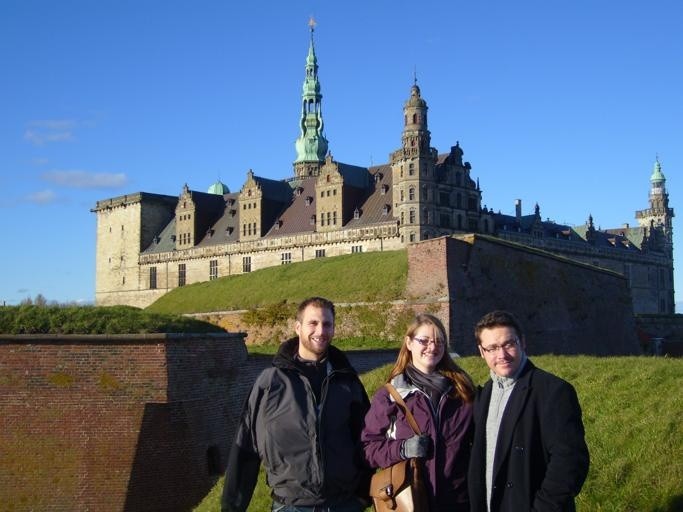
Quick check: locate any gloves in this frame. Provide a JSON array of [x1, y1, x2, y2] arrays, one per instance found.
[[401, 432, 431, 460]]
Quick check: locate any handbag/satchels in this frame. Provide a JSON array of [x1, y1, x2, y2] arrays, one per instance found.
[[369, 456, 431, 512]]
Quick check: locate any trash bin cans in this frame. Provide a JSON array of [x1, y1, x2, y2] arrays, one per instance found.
[[649, 338, 665, 356]]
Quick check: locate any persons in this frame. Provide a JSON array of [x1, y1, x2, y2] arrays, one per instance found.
[[221, 294, 374, 511], [466, 311, 590, 512], [360, 312, 475, 511]]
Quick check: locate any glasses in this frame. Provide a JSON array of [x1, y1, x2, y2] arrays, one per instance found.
[[413, 336, 444, 346], [479, 338, 520, 353]]
